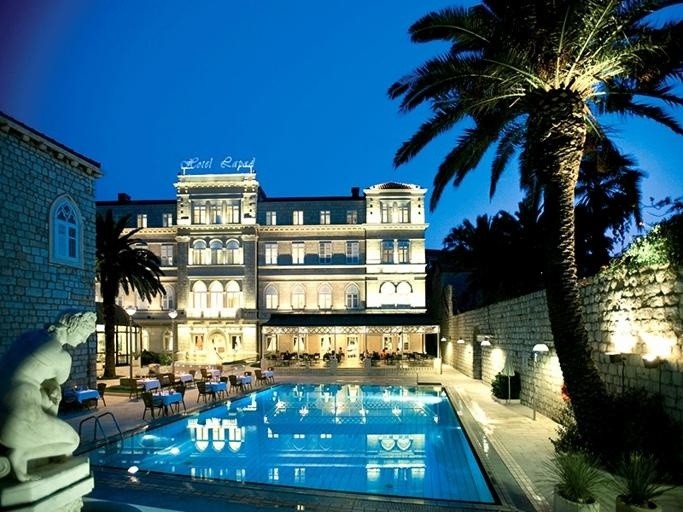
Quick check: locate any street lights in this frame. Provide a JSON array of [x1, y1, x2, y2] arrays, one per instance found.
[[168, 309, 177, 374], [126, 306, 136, 388]]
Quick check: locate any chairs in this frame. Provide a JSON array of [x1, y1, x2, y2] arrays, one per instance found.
[[61, 367, 275, 419]]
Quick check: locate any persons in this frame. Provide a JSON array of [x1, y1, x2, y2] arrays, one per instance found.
[[1, 309, 98, 484]]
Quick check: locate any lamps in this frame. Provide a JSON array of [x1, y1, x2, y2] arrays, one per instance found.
[[532, 340, 549, 351], [480, 337, 490, 346], [457, 337, 464, 343], [441, 335, 451, 341]]
